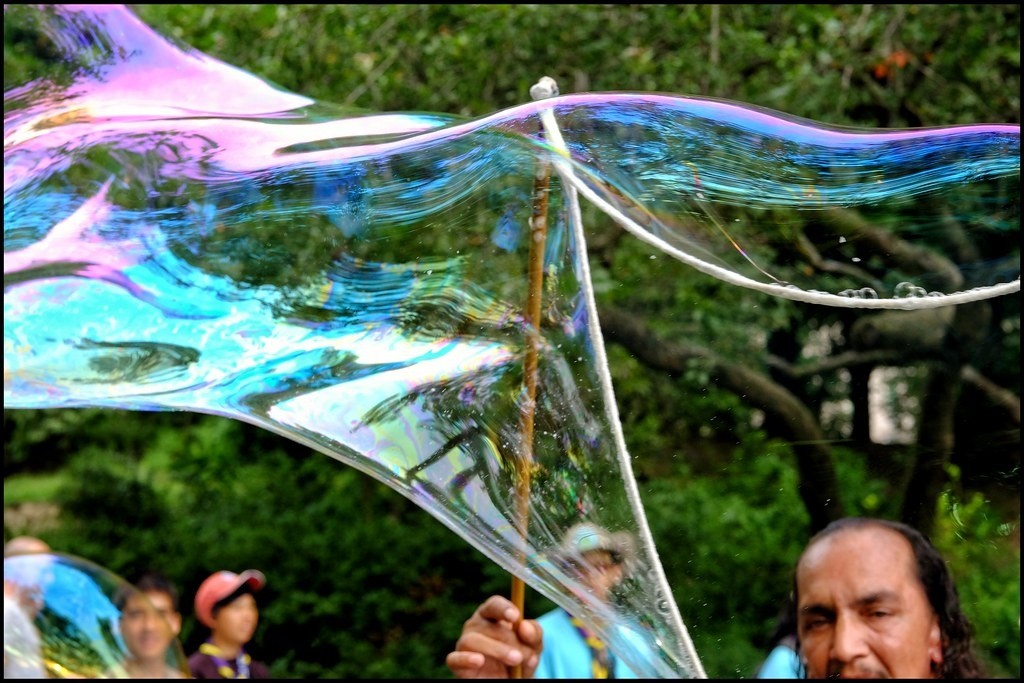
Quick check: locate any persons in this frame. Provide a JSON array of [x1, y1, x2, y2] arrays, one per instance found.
[[97, 578, 193, 678], [446, 518, 980, 679], [4, 535, 56, 679], [183, 571, 269, 678], [529, 525, 680, 679], [756, 633, 805, 679]]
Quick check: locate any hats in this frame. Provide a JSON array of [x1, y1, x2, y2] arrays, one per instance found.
[[551, 523, 635, 579], [195, 569, 267, 628]]
